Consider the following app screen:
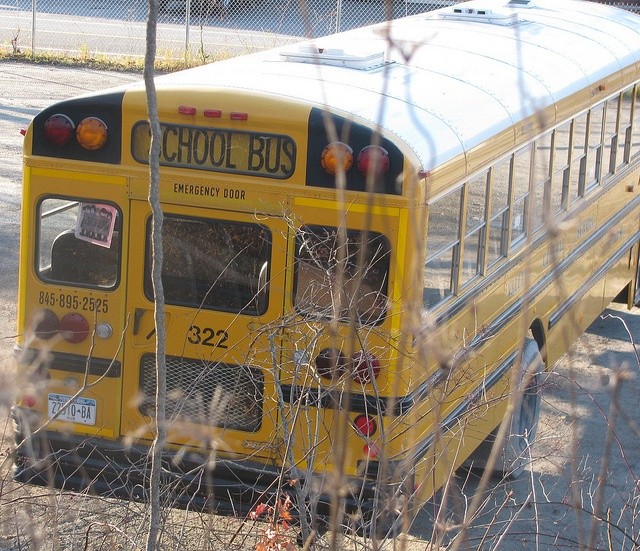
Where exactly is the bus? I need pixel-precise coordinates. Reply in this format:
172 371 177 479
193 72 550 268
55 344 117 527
10 0 639 536
